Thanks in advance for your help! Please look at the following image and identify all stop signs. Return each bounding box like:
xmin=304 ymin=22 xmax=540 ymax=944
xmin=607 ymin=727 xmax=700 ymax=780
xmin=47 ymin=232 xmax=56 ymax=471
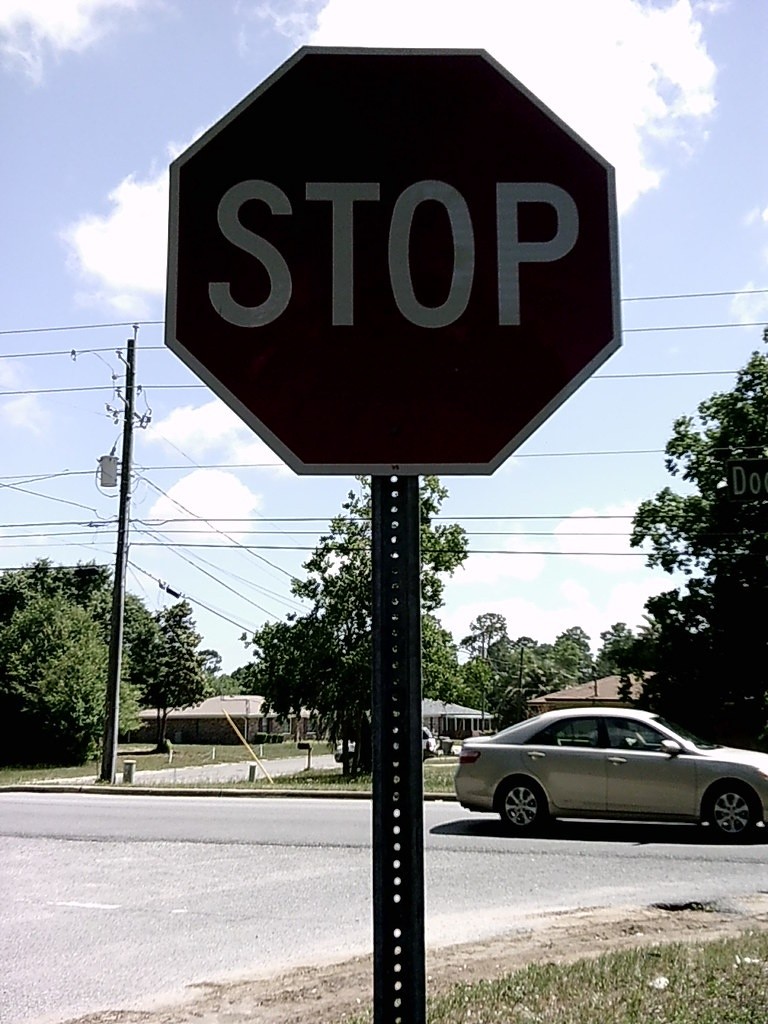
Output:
xmin=163 ymin=45 xmax=621 ymax=476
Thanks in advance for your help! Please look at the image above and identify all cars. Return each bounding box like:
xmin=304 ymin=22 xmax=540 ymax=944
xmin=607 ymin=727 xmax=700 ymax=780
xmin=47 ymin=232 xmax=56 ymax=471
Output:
xmin=454 ymin=707 xmax=768 ymax=841
